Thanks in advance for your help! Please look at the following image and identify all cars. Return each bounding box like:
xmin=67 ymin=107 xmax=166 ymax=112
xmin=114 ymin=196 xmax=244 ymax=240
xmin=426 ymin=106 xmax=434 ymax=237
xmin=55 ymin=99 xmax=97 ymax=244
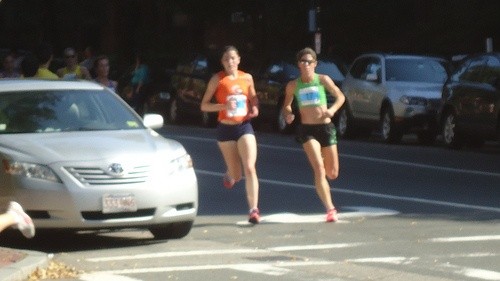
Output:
xmin=436 ymin=53 xmax=500 ymax=148
xmin=0 ymin=79 xmax=197 ymax=240
xmin=137 ymin=46 xmax=344 ymax=135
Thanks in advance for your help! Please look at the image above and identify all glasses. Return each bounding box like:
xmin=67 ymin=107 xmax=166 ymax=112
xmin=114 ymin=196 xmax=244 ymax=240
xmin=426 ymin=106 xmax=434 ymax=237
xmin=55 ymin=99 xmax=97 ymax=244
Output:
xmin=299 ymin=59 xmax=315 ymax=66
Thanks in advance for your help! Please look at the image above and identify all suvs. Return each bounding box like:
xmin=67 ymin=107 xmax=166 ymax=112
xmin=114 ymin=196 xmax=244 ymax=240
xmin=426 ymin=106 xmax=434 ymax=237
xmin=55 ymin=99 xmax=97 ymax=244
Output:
xmin=338 ymin=50 xmax=458 ymax=143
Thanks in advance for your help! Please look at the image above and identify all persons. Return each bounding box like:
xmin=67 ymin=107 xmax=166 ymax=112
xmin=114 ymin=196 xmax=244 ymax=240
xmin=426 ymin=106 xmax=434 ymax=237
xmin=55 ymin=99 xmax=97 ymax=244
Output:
xmin=0 ymin=44 xmax=200 ymax=122
xmin=284 ymin=48 xmax=345 ymax=221
xmin=201 ymin=46 xmax=259 ymax=224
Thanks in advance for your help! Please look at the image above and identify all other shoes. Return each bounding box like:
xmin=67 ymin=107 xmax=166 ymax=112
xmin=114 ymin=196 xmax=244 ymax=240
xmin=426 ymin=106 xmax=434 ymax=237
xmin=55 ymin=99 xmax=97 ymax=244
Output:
xmin=8 ymin=201 xmax=36 ymax=239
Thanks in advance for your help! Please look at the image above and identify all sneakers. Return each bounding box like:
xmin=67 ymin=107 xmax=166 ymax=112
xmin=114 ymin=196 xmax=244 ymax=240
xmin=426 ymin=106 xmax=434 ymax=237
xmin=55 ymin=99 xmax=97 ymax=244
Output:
xmin=326 ymin=207 xmax=338 ymax=221
xmin=224 ymin=174 xmax=236 ymax=189
xmin=248 ymin=208 xmax=259 ymax=222
